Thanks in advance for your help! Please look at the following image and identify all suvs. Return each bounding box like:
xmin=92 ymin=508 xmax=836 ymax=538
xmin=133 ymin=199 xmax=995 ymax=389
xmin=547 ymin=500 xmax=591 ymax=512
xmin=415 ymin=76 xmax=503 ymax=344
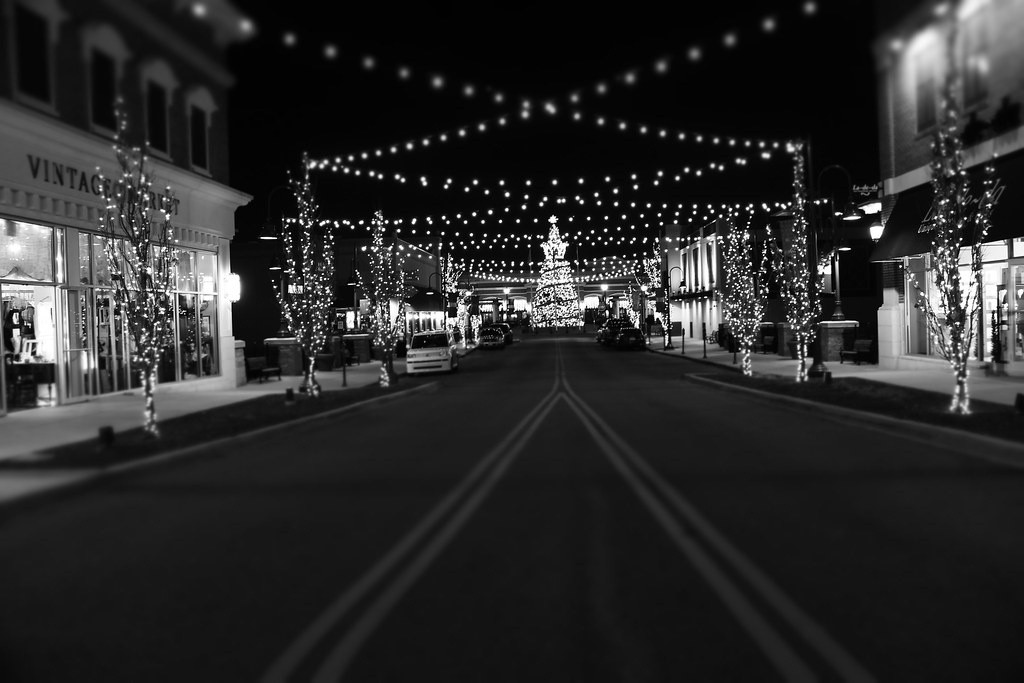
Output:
xmin=597 ymin=319 xmax=635 ymax=345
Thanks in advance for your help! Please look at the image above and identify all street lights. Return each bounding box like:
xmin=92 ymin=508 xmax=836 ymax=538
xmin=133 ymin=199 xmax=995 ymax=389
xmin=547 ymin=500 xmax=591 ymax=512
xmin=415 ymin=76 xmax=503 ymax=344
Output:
xmin=807 ymin=164 xmax=883 ymax=376
xmin=665 ymin=266 xmax=687 ymax=350
xmin=426 ymin=272 xmax=458 ymax=334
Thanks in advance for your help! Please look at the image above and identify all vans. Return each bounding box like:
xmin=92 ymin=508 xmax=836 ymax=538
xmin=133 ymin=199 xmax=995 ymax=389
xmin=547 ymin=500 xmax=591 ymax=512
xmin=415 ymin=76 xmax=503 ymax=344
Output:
xmin=406 ymin=332 xmax=460 ymax=373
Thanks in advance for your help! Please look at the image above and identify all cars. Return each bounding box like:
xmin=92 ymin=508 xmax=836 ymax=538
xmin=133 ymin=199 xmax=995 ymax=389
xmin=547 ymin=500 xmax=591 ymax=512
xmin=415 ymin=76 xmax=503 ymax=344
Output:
xmin=490 ymin=322 xmax=514 ymax=344
xmin=617 ymin=328 xmax=647 ymax=349
xmin=480 ymin=328 xmax=505 ymax=346
